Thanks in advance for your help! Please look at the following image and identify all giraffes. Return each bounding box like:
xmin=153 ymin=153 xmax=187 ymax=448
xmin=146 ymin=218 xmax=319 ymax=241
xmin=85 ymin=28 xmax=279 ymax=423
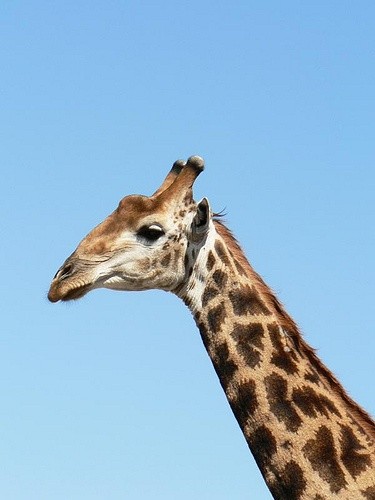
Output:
xmin=45 ymin=151 xmax=375 ymax=499
